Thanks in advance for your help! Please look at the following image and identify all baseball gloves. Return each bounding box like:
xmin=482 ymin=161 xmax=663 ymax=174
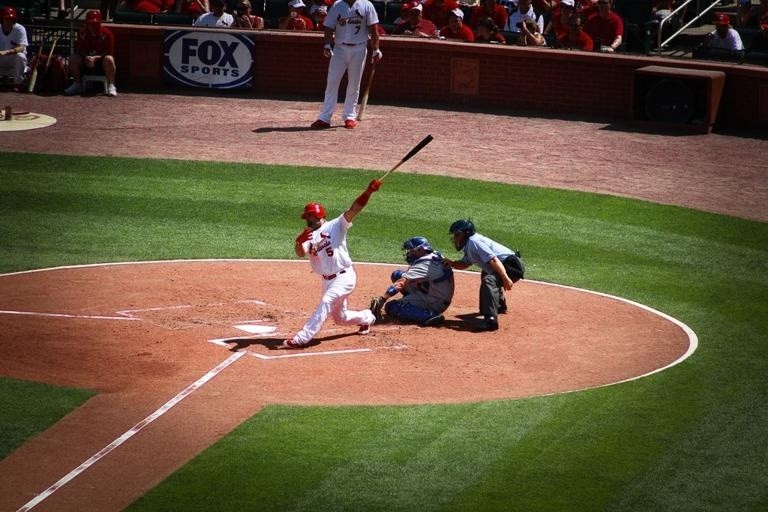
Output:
xmin=369 ymin=298 xmax=386 ymax=322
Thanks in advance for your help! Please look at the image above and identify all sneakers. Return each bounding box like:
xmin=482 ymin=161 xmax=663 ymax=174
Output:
xmin=357 ymin=317 xmax=376 ymax=335
xmin=423 ymin=312 xmax=443 ymax=326
xmin=345 ymin=119 xmax=355 ymax=129
xmin=497 ymin=304 xmax=506 ymax=314
xmin=64 ymin=83 xmax=81 ymax=94
xmin=107 ymin=85 xmax=117 ymax=97
xmin=282 ymin=339 xmax=298 ymax=349
xmin=310 ymin=119 xmax=330 ymax=130
xmin=470 ymin=317 xmax=497 ymax=332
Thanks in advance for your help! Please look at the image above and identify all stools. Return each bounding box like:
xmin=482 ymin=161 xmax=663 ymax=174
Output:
xmin=81 ymin=75 xmax=109 ymax=95
xmin=0 ymin=66 xmax=32 ymax=92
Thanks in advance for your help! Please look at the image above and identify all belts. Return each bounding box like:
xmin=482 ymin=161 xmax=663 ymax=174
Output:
xmin=323 ymin=270 xmax=344 ymax=280
xmin=342 ymin=42 xmax=364 ymax=46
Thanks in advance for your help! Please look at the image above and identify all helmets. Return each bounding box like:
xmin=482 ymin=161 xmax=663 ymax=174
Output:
xmin=449 ymin=219 xmax=475 ymax=251
xmin=300 ymin=202 xmax=325 ymax=219
xmin=399 ymin=236 xmax=433 ymax=265
xmin=86 ymin=10 xmax=102 ymax=24
xmin=0 ymin=5 xmax=17 ymax=20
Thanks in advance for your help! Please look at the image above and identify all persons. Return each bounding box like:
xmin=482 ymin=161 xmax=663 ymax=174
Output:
xmin=119 ymin=1 xmax=331 ymax=32
xmin=0 ymin=6 xmax=30 ymax=93
xmin=282 ymin=179 xmax=382 ymax=349
xmin=696 ymin=1 xmax=767 ymax=64
xmin=62 ymin=11 xmax=118 ymax=97
xmin=367 ymin=235 xmax=456 ymax=327
xmin=310 ymin=0 xmax=383 ymax=131
xmin=441 ymin=218 xmax=525 ymax=332
xmin=373 ymin=1 xmax=692 ymax=54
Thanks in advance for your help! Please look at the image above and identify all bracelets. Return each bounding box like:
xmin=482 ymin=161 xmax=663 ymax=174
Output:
xmin=386 ymin=285 xmax=398 ymax=297
xmin=324 ymin=44 xmax=331 ymax=49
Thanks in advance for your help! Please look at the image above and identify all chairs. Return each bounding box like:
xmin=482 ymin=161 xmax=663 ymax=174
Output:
xmin=745 ymin=52 xmax=768 ymax=67
xmin=152 ymin=14 xmax=193 ymax=25
xmin=692 ymin=43 xmax=712 ymax=60
xmin=381 ymin=2 xmax=402 ymax=33
xmin=541 ymin=34 xmax=557 ymax=46
xmin=373 ymin=2 xmax=385 ymax=23
xmin=115 ymin=11 xmax=151 ymax=24
xmin=500 ymin=30 xmax=520 ymax=44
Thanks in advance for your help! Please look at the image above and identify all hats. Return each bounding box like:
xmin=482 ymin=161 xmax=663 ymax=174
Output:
xmin=287 ymin=0 xmax=306 ymax=8
xmin=516 ymin=18 xmax=537 ymax=34
xmin=559 ymin=0 xmax=574 ymax=7
xmin=450 ymin=7 xmax=464 ymax=20
xmin=711 ymin=14 xmax=729 ymax=25
xmin=411 ymin=3 xmax=423 ymax=13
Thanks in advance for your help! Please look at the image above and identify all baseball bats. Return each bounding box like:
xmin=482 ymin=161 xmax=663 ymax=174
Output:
xmin=358 ymin=57 xmax=380 ymax=122
xmin=26 ymin=36 xmax=60 ymax=94
xmin=370 ymin=134 xmax=434 ymax=190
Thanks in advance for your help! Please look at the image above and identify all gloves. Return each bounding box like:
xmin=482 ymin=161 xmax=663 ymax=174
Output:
xmin=370 ymin=48 xmax=382 ymax=64
xmin=367 ymin=179 xmax=380 ymax=192
xmin=323 ymin=43 xmax=333 ymax=58
